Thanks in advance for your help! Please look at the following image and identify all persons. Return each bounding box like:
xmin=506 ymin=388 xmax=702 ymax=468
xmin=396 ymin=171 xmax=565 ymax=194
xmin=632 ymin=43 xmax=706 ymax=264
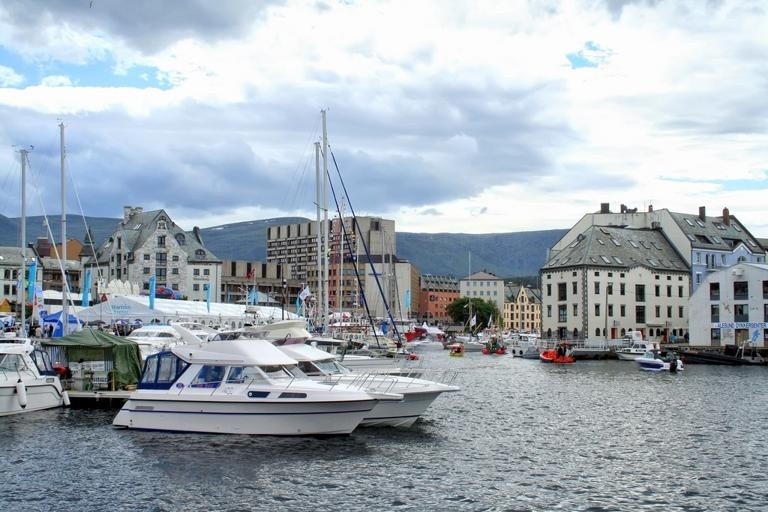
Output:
xmin=395 ymin=341 xmax=401 ymax=352
xmin=555 ymin=344 xmax=568 ymax=359
xmin=0 ymin=327 xmax=5 ymax=337
xmin=33 ymin=323 xmax=53 ymax=338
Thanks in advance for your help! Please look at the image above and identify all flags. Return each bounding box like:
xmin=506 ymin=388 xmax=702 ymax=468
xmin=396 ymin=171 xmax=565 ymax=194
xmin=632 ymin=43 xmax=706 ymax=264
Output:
xmin=471 ymin=315 xmax=476 ymax=327
xmin=486 ymin=314 xmax=492 ymax=327
xmin=298 ymin=286 xmax=310 ymax=301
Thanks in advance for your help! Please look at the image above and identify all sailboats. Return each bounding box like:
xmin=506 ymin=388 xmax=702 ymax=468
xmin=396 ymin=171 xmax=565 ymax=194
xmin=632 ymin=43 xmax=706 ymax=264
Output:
xmin=0 ymin=144 xmax=71 ymax=418
xmin=464 ymin=298 xmax=484 ymax=351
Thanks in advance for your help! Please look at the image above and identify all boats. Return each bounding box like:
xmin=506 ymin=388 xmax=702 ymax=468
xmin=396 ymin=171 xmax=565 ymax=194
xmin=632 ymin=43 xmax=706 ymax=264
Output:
xmin=0 ymin=107 xmax=461 ymax=419
xmin=110 ymin=335 xmax=404 ymax=441
xmin=447 ymin=343 xmax=465 ymax=356
xmin=275 ymin=340 xmax=463 ymax=429
xmin=482 ymin=330 xmax=763 ymax=372
